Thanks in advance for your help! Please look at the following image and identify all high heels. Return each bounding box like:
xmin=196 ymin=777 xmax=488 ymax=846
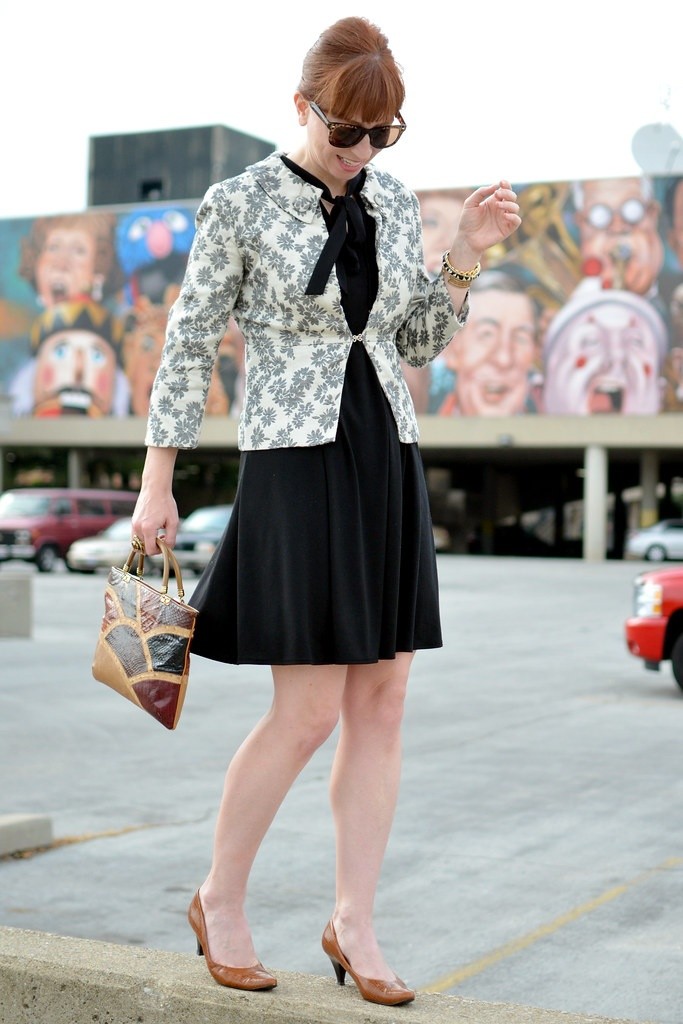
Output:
xmin=321 ymin=918 xmax=416 ymax=1005
xmin=188 ymin=889 xmax=278 ymax=991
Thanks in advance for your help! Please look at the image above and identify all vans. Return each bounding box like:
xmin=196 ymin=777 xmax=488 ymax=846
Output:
xmin=1 ymin=489 xmax=141 ymax=572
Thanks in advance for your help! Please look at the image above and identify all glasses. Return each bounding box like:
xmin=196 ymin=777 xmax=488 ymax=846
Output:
xmin=308 ymin=95 xmax=406 ymax=149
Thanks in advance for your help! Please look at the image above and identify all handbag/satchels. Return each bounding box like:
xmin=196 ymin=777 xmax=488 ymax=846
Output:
xmin=91 ymin=542 xmax=199 ymax=731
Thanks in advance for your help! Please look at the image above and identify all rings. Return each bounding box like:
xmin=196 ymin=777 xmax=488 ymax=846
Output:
xmin=132 ymin=534 xmax=145 ymax=555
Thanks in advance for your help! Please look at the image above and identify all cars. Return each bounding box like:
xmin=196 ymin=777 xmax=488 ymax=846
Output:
xmin=628 ymin=520 xmax=683 ymax=562
xmin=151 ymin=507 xmax=236 ymax=576
xmin=628 ymin=560 xmax=682 ymax=693
xmin=66 ymin=515 xmax=150 ymax=575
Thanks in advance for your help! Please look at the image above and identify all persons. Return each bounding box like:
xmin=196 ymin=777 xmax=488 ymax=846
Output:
xmin=132 ymin=18 xmax=520 ymax=1003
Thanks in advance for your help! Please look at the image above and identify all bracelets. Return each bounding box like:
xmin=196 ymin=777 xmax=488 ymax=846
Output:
xmin=442 ymin=250 xmax=481 ymax=287
